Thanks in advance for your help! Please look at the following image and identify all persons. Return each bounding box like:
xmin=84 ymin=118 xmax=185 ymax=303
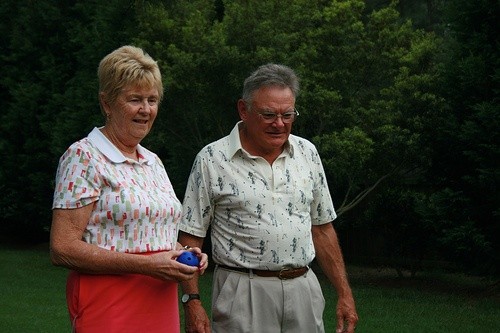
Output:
xmin=50 ymin=46 xmax=208 ymax=332
xmin=178 ymin=62 xmax=358 ymax=333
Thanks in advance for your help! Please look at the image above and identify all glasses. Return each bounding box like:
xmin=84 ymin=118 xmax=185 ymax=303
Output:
xmin=247 ymin=108 xmax=299 ymax=124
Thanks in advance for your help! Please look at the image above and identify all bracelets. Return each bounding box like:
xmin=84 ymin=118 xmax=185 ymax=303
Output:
xmin=179 ymin=246 xmax=192 ymax=250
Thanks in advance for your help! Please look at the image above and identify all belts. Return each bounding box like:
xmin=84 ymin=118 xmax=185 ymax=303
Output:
xmin=219 ymin=264 xmax=309 ymax=280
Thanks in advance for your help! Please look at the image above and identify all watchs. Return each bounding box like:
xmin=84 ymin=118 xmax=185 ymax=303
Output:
xmin=181 ymin=294 xmax=200 ymax=304
